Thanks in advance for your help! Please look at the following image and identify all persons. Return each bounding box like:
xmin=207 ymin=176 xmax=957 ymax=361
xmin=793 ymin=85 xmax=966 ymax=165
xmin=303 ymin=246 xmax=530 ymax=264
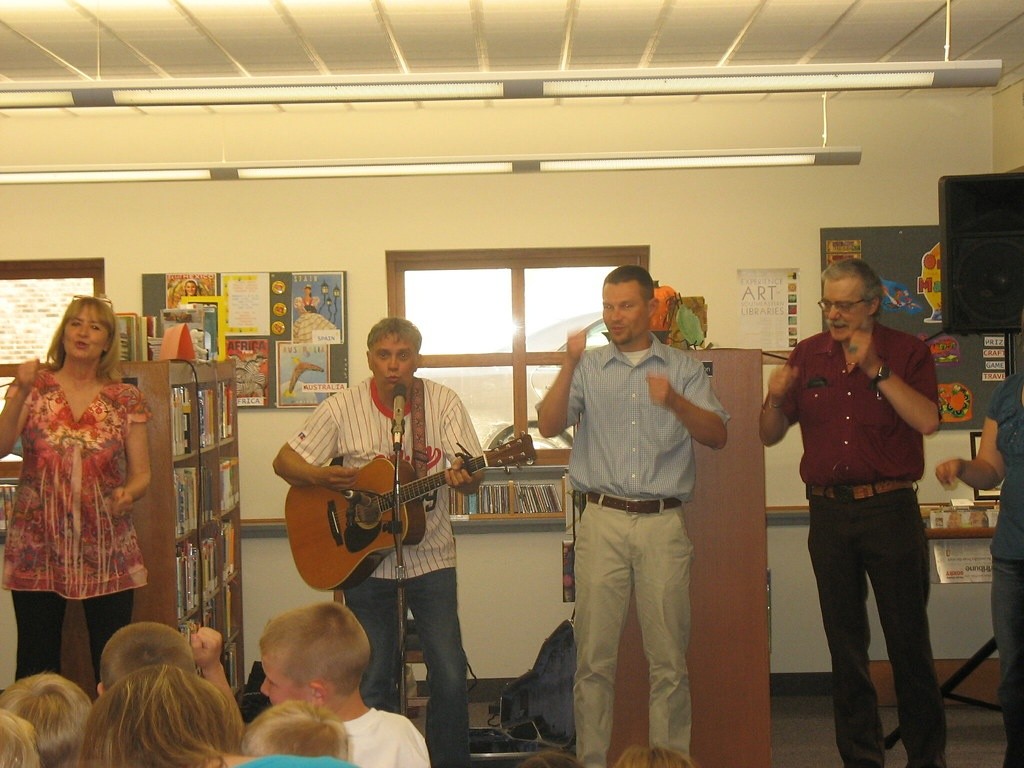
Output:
xmin=517 ymin=748 xmax=586 ymax=768
xmin=0 ymin=602 xmax=431 ymax=768
xmin=613 ymin=743 xmax=692 ymax=768
xmin=760 ymin=258 xmax=944 ymax=768
xmin=0 ymin=296 xmax=153 ymax=695
xmin=935 ymin=373 xmax=1024 ymax=768
xmin=535 ymin=265 xmax=731 ymax=759
xmin=272 ymin=319 xmax=487 ymax=768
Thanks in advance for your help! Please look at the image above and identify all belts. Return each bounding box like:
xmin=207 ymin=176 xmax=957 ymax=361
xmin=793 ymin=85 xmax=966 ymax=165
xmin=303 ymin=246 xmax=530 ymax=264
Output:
xmin=807 ymin=478 xmax=915 ymax=499
xmin=587 ymin=491 xmax=682 ymax=513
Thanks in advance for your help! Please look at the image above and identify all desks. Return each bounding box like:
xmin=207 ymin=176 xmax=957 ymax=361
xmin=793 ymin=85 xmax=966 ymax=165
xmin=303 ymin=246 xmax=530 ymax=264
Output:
xmin=884 ymin=526 xmax=1001 ymax=751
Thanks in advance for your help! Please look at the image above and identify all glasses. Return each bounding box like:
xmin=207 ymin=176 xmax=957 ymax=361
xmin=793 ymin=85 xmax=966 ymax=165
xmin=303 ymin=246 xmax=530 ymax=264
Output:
xmin=819 ymin=294 xmax=878 ymax=313
xmin=72 ymin=295 xmax=113 ymax=311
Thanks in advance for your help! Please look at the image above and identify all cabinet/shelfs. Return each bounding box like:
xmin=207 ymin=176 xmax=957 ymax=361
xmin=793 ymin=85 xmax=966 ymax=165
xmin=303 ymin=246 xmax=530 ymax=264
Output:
xmin=607 ymin=349 xmax=772 ymax=767
xmin=105 ymin=360 xmax=246 ymax=702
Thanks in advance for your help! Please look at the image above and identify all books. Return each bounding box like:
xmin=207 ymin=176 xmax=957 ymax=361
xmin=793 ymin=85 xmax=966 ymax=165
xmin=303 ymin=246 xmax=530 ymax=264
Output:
xmin=115 ymin=312 xmax=162 ymax=361
xmin=446 ymin=483 xmax=563 ymax=515
xmin=169 ymin=386 xmax=238 ymax=694
xmin=0 ymin=483 xmax=18 ymax=528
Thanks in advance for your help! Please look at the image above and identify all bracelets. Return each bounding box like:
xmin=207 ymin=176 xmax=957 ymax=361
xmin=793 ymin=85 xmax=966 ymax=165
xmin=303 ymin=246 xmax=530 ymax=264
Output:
xmin=767 ymin=399 xmax=784 ymax=408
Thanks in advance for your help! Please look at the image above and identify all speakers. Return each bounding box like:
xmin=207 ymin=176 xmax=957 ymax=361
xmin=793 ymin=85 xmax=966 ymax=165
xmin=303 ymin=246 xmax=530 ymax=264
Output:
xmin=939 ymin=172 xmax=1023 ymax=334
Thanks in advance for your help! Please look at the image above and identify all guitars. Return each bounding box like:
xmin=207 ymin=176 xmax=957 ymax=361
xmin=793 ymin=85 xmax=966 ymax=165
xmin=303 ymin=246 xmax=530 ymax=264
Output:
xmin=285 ymin=432 xmax=537 ymax=591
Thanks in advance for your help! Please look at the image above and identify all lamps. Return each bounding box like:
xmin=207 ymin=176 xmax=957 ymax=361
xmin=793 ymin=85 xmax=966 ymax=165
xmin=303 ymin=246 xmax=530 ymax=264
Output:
xmin=0 ymin=1 xmax=1003 ymax=185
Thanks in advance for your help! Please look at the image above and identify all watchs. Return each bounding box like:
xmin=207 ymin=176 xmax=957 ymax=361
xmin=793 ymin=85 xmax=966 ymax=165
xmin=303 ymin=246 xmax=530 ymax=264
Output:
xmin=871 ymin=364 xmax=891 ymax=384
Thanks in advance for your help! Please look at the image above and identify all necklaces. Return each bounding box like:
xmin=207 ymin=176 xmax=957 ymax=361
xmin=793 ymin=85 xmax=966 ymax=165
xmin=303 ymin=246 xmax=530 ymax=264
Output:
xmin=846 ymin=362 xmax=855 ymax=365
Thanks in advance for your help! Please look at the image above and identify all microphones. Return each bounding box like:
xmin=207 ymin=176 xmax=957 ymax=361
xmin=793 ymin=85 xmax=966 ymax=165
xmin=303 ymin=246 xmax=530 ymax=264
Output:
xmin=390 ymin=382 xmax=406 ymax=452
